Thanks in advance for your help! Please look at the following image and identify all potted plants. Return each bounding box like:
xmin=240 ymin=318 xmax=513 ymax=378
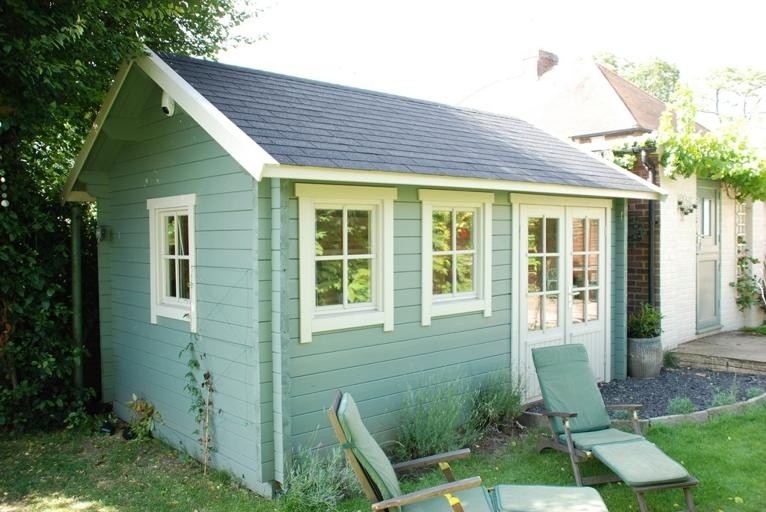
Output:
xmin=727 ymin=255 xmax=766 ymax=329
xmin=628 ymin=301 xmax=667 ymax=378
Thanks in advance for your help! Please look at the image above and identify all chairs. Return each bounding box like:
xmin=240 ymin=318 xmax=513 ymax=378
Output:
xmin=327 ymin=388 xmax=609 ymax=512
xmin=531 ymin=342 xmax=700 ymax=511
